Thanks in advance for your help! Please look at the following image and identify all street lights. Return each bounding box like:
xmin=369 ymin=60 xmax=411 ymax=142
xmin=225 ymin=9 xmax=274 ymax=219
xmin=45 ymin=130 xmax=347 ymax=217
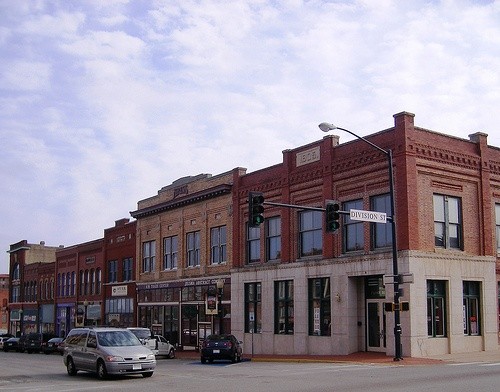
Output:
xmin=84 ymin=298 xmax=89 ymax=326
xmin=216 ymin=278 xmax=224 ymax=336
xmin=317 ymin=121 xmax=404 ymax=362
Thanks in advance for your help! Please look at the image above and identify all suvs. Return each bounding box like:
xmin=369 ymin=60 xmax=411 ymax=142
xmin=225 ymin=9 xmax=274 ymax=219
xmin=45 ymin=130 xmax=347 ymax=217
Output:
xmin=126 ymin=326 xmax=158 ymax=356
xmin=24 ymin=332 xmax=59 ymax=354
xmin=63 ymin=326 xmax=157 ymax=380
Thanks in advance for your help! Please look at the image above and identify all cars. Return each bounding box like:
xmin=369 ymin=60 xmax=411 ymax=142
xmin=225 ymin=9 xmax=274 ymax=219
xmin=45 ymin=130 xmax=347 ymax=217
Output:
xmin=200 ymin=333 xmax=243 ymax=364
xmin=153 ymin=334 xmax=176 ymax=360
xmin=44 ymin=338 xmax=65 ymax=356
xmin=0 ymin=337 xmax=25 ymax=353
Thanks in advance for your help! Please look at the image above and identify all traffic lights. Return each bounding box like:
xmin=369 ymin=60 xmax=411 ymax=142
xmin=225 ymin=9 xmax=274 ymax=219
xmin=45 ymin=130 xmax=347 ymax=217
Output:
xmin=385 ymin=302 xmax=395 ymax=312
xmin=400 ymin=300 xmax=409 ymax=312
xmin=249 ymin=191 xmax=264 ymax=228
xmin=324 ymin=199 xmax=340 ymax=235
